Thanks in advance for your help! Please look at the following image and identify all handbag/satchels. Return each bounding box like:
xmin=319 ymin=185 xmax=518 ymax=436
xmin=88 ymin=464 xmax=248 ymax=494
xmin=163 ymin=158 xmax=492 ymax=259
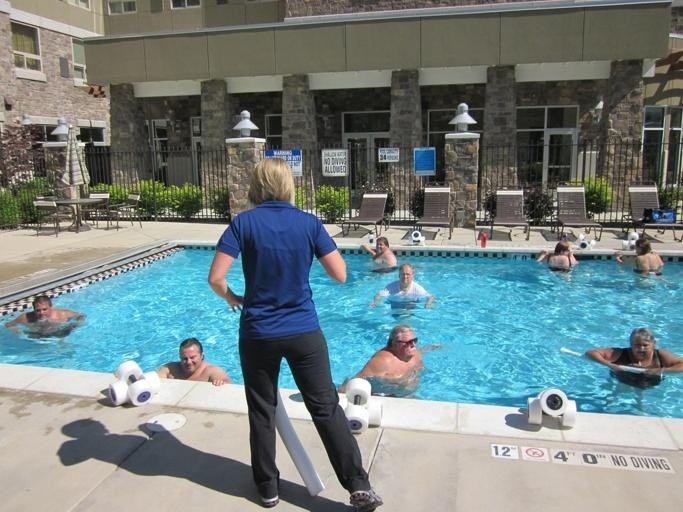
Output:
xmin=642 ymin=208 xmax=676 ymax=224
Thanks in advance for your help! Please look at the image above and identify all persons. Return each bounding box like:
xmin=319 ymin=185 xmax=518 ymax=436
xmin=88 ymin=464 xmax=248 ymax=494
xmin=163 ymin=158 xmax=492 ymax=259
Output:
xmin=586 ymin=328 xmax=683 ymax=375
xmin=4 ymin=295 xmax=84 ymax=339
xmin=368 ymin=263 xmax=433 ymax=318
xmin=208 ymin=157 xmax=383 ymax=511
xmin=537 ymin=241 xmax=578 ymax=281
xmin=615 ymin=238 xmax=665 ymax=279
xmin=361 ymin=236 xmax=398 ymax=273
xmin=155 ymin=338 xmax=232 ymax=386
xmin=334 ymin=325 xmax=440 ymax=399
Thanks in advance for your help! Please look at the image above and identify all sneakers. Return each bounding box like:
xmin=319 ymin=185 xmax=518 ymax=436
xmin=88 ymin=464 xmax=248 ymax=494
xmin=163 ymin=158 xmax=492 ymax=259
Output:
xmin=350 ymin=487 xmax=383 ymax=512
xmin=261 ymin=494 xmax=279 ymax=507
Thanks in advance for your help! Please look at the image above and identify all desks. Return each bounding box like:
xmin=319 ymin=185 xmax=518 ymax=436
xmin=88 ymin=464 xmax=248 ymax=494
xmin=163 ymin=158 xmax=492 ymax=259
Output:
xmin=55 ymin=198 xmax=103 ymax=232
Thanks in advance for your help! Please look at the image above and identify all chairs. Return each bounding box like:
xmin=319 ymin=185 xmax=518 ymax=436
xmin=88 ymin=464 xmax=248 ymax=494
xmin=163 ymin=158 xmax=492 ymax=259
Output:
xmin=551 ymin=181 xmax=603 ymax=241
xmin=622 ymin=180 xmax=683 ymax=242
xmin=35 ymin=194 xmax=57 ymax=224
xmin=416 ymin=182 xmax=454 ymax=240
xmin=342 ymin=190 xmax=390 ymax=238
xmin=85 ymin=192 xmax=112 ymax=229
xmin=110 ymin=193 xmax=142 ymax=231
xmin=490 ymin=185 xmax=529 ymax=241
xmin=33 ymin=200 xmax=60 ymax=237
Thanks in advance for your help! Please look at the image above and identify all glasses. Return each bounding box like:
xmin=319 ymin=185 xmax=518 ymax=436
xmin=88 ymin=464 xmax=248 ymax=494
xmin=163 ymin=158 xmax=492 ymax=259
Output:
xmin=398 ymin=338 xmax=418 ymax=345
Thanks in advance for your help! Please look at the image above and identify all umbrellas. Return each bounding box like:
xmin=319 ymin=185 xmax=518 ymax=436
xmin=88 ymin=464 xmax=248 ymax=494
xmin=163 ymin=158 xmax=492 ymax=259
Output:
xmin=61 ymin=125 xmax=91 ymax=224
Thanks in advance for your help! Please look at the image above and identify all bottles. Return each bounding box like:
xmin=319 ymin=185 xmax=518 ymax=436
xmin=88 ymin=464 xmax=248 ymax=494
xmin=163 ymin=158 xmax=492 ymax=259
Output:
xmin=481 ymin=231 xmax=487 ymax=248
